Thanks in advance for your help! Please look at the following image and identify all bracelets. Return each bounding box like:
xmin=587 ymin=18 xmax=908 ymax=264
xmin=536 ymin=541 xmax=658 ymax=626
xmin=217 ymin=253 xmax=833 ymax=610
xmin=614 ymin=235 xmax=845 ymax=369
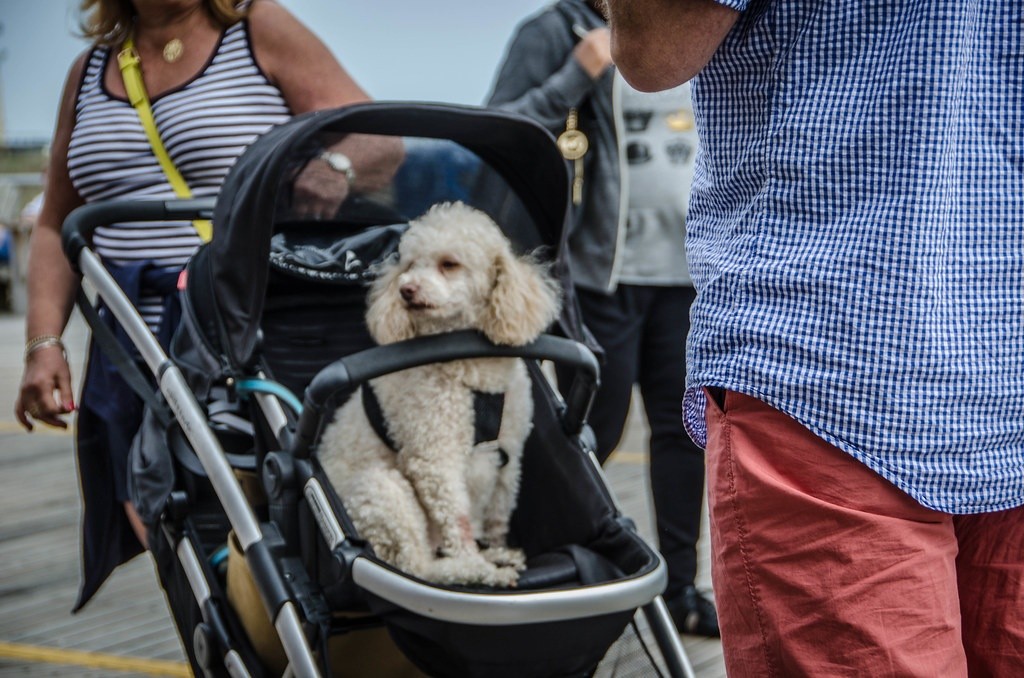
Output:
xmin=23 ymin=333 xmax=67 ymax=361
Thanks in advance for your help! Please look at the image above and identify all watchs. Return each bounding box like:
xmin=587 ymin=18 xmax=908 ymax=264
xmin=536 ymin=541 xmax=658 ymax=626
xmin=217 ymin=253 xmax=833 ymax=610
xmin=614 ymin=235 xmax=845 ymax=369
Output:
xmin=319 ymin=153 xmax=356 ymax=183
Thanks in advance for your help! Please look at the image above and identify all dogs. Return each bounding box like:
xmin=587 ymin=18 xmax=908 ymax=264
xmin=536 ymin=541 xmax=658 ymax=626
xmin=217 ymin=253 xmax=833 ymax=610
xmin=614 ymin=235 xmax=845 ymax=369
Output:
xmin=313 ymin=196 xmax=565 ymax=592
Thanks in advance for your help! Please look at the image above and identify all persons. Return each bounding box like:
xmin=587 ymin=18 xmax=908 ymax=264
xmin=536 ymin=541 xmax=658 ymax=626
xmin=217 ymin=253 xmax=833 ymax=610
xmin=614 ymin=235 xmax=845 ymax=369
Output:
xmin=483 ymin=0 xmax=720 ymax=638
xmin=606 ymin=0 xmax=1023 ymax=678
xmin=14 ymin=0 xmax=404 ymax=614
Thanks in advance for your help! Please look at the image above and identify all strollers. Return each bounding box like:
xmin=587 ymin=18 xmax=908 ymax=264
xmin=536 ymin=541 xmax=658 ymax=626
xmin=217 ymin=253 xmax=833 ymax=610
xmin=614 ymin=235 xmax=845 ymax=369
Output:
xmin=61 ymin=101 xmax=694 ymax=678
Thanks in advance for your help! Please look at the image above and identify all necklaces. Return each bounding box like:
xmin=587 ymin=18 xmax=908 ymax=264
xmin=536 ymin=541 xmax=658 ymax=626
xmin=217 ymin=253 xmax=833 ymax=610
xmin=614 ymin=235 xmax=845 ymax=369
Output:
xmin=160 ymin=37 xmax=183 ymax=64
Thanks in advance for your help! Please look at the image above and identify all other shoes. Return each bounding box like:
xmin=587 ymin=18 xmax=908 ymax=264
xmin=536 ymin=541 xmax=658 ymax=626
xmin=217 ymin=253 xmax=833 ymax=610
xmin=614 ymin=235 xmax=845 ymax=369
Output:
xmin=667 ymin=586 xmax=718 ymax=638
